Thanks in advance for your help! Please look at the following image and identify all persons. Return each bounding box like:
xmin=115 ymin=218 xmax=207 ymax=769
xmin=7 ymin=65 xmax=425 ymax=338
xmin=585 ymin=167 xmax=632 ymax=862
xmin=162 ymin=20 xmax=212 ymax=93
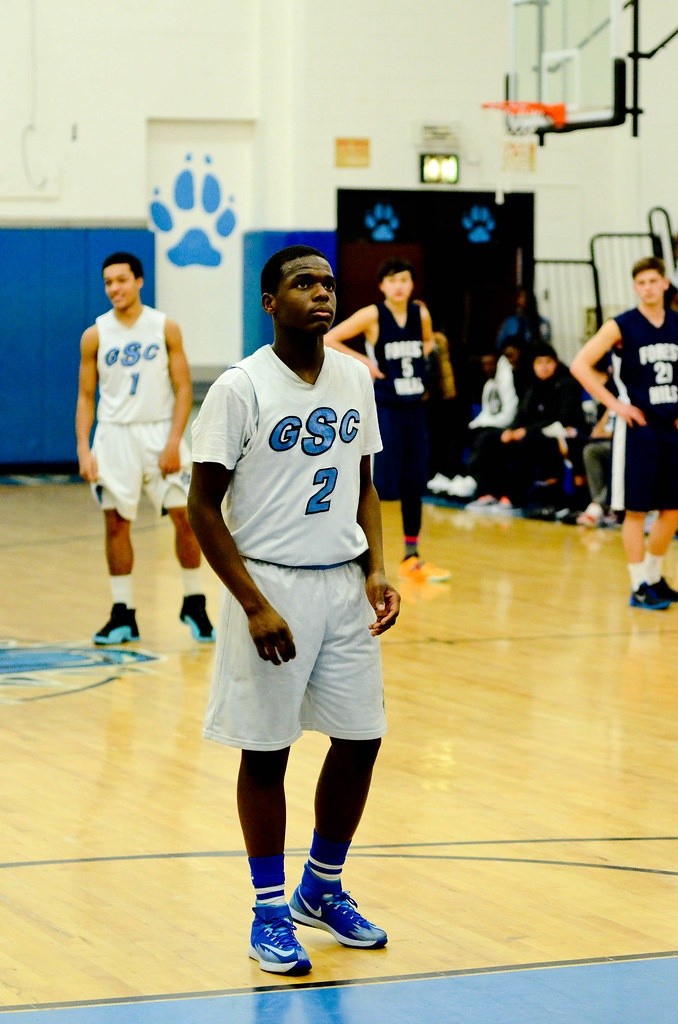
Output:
xmin=427 ymin=284 xmax=678 ymax=540
xmin=322 ymin=259 xmax=450 ymax=583
xmin=187 ymin=246 xmax=401 ymax=974
xmin=570 ymin=258 xmax=678 ymax=610
xmin=76 ymin=253 xmax=216 ymax=644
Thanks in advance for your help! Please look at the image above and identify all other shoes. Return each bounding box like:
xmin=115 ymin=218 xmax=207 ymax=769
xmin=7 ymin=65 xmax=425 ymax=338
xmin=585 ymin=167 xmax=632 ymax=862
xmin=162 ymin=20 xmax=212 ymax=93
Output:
xmin=576 ymin=501 xmax=603 ymax=529
xmin=464 ymin=496 xmax=513 ymax=515
xmin=398 ymin=554 xmax=451 ymax=583
xmin=427 ymin=472 xmax=479 ymax=498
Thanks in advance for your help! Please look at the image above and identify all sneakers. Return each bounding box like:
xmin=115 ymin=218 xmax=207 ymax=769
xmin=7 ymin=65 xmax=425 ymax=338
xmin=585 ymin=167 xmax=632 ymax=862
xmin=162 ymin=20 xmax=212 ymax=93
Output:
xmin=180 ymin=593 xmax=216 ymax=643
xmin=630 ymin=576 xmax=678 ymax=611
xmin=248 ymin=899 xmax=312 ymax=977
xmin=92 ymin=604 xmax=141 ymax=644
xmin=289 ymin=882 xmax=389 ymax=950
